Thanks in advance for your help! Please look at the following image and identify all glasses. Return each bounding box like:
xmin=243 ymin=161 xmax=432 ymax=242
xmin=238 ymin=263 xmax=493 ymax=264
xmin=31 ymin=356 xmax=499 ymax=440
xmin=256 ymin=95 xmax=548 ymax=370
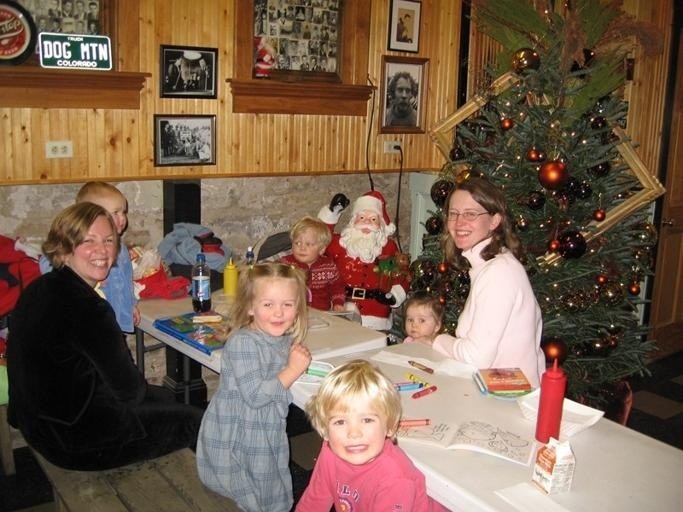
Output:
xmin=445 ymin=208 xmax=493 ymax=221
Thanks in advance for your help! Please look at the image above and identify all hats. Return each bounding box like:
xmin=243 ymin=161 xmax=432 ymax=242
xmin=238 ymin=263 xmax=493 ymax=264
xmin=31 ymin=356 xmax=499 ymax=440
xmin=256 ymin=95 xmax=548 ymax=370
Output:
xmin=354 ymin=191 xmax=397 ymax=235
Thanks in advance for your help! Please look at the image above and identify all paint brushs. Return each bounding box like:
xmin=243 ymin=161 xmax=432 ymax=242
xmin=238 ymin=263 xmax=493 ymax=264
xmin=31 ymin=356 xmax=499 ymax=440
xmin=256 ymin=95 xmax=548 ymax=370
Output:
xmin=392 ymin=381 xmax=416 ymax=387
xmin=411 ymin=385 xmax=438 ymax=398
xmin=303 ymin=368 xmax=328 ymax=378
xmin=394 ymin=383 xmax=423 ymax=392
xmin=408 ymin=360 xmax=434 ymax=374
xmin=398 ymin=419 xmax=430 ymax=426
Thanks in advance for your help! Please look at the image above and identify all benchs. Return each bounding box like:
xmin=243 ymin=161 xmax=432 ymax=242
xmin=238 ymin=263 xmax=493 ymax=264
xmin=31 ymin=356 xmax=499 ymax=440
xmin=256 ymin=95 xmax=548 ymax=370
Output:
xmin=29 ymin=446 xmax=247 ymax=512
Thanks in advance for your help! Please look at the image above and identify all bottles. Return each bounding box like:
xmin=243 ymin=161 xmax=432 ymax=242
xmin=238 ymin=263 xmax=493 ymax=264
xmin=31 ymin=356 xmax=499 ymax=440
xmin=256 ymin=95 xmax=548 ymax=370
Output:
xmin=535 ymin=359 xmax=567 ymax=444
xmin=246 ymin=247 xmax=253 ymax=265
xmin=191 ymin=254 xmax=211 ymax=313
xmin=222 ymin=255 xmax=238 ymax=296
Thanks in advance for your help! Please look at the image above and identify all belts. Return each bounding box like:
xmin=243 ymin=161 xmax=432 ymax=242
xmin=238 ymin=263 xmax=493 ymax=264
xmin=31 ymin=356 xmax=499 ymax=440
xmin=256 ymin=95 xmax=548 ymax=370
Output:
xmin=344 ymin=286 xmax=383 ymax=300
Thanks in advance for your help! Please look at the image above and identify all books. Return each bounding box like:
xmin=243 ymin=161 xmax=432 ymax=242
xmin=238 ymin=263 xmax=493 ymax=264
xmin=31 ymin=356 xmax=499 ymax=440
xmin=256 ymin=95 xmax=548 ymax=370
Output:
xmin=478 ymin=368 xmax=532 ymax=391
xmin=397 ymin=416 xmax=535 ymax=466
xmin=473 ymin=372 xmax=529 ymax=395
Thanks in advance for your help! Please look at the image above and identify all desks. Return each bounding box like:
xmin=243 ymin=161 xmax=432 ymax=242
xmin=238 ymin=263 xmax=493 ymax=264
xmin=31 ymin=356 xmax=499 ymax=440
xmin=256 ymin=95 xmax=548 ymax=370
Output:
xmin=289 ymin=341 xmax=683 ymax=512
xmin=134 ymin=287 xmax=387 ymax=406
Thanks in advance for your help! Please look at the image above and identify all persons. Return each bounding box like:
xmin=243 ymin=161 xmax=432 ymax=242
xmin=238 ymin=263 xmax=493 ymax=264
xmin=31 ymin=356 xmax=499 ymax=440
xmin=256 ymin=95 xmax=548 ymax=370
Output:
xmin=386 ymin=72 xmax=418 ymax=127
xmin=197 ymin=262 xmax=314 ymax=512
xmin=88 ymin=22 xmax=98 ymax=35
xmin=50 ymin=19 xmax=62 ymax=32
xmin=62 ymin=1 xmax=73 ymax=16
xmin=397 ymin=15 xmax=413 ymax=43
xmin=48 ymin=0 xmax=61 ymax=17
xmin=75 ymin=1 xmax=86 ymax=19
xmin=87 ymin=2 xmax=99 ymax=20
xmin=268 ymin=0 xmax=339 ymax=72
xmin=165 ymin=57 xmax=213 ymax=92
xmin=162 ymin=123 xmax=211 ymax=160
xmin=403 ymin=294 xmax=444 ymax=343
xmin=37 ymin=17 xmax=46 ymax=33
xmin=295 ymin=360 xmax=450 ymax=511
xmin=274 ymin=217 xmax=346 ymax=312
xmin=40 ymin=181 xmax=141 ymax=332
xmin=253 ymin=37 xmax=275 ymax=79
xmin=413 ymin=177 xmax=545 ymax=388
xmin=76 ymin=22 xmax=86 ymax=35
xmin=317 ymin=191 xmax=414 ymax=330
xmin=7 ymin=203 xmax=205 ymax=471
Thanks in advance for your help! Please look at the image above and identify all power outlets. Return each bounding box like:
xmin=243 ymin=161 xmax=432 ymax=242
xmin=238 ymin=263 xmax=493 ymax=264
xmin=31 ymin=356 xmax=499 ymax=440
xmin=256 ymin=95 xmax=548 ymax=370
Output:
xmin=45 ymin=141 xmax=73 ymax=159
xmin=383 ymin=142 xmax=402 ymax=154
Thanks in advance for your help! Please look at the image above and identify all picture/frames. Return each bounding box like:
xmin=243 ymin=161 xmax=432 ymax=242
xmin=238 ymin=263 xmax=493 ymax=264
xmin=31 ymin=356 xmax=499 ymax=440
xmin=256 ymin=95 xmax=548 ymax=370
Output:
xmin=387 ymin=0 xmax=421 ymax=53
xmin=378 ymin=54 xmax=430 ymax=134
xmin=154 ymin=114 xmax=216 ymax=166
xmin=159 ymin=44 xmax=219 ymax=99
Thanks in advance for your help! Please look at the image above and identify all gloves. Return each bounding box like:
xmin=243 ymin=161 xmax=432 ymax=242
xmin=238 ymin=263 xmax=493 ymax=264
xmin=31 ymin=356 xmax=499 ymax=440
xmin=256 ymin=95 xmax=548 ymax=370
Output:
xmin=374 ymin=289 xmax=396 ymax=306
xmin=329 ymin=192 xmax=351 ymax=213
